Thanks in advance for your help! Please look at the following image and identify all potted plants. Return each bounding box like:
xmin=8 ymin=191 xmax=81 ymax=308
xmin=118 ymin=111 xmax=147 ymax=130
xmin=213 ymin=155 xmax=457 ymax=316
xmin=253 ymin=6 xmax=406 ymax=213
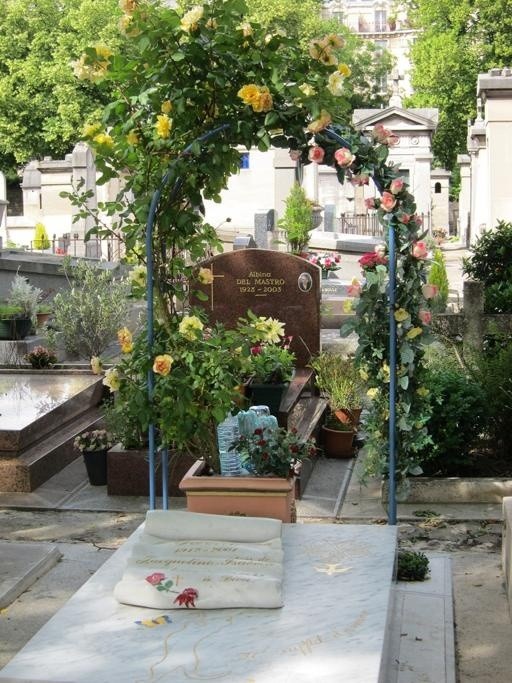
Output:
xmin=302 ymin=340 xmax=374 ymax=459
xmin=0 ymin=264 xmax=53 ymax=340
xmin=72 ymin=428 xmax=109 ymax=485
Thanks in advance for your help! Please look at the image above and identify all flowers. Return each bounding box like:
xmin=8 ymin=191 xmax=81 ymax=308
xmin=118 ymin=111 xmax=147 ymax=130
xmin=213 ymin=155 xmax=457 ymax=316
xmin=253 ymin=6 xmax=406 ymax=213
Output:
xmin=304 ymin=250 xmax=343 ymax=271
xmin=227 ymin=425 xmax=325 ymax=478
